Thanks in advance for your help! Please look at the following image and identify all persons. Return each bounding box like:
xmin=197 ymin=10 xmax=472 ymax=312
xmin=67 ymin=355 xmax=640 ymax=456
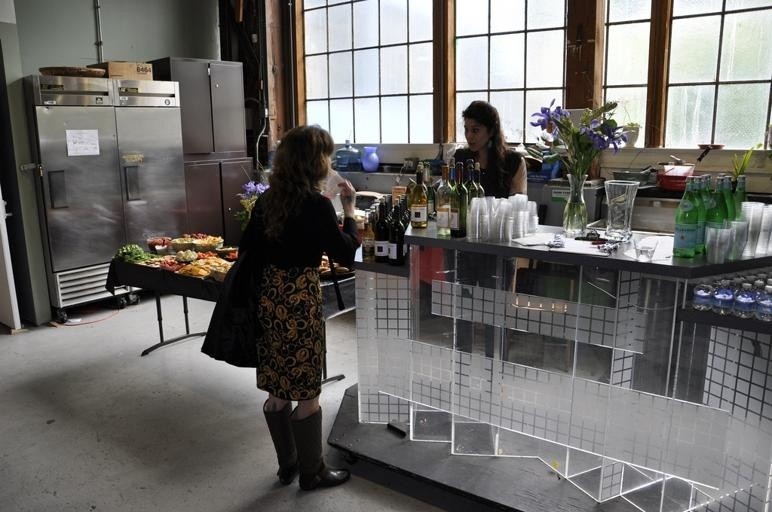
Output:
xmin=201 ymin=126 xmax=360 ymax=492
xmin=450 ymin=101 xmax=527 ymax=394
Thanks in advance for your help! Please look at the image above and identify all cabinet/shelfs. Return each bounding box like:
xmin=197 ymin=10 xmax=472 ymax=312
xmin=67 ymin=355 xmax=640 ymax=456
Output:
xmin=185 ymin=157 xmax=253 ymax=244
xmin=147 ymin=55 xmax=247 ymax=160
xmin=328 ymin=205 xmax=772 ymax=511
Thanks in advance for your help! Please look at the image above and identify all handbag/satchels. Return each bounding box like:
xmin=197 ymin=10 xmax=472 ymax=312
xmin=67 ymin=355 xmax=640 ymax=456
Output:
xmin=199 ymin=260 xmax=261 ymax=369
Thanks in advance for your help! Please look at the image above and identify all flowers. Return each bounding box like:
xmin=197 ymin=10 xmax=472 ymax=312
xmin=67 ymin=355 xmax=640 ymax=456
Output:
xmin=525 ymin=99 xmax=641 ymax=173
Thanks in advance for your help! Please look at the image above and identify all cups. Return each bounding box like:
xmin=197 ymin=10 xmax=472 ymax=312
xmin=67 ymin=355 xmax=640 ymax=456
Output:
xmin=705 ymin=202 xmax=772 ymax=264
xmin=605 ymin=180 xmax=640 ymax=242
xmin=467 ymin=194 xmax=538 ymax=242
xmin=634 ymin=237 xmax=658 ymax=262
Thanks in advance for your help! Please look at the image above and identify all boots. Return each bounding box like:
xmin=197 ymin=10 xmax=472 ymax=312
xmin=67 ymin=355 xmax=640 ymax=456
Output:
xmin=262 ymin=398 xmax=300 ymax=487
xmin=288 ymin=404 xmax=351 ymax=491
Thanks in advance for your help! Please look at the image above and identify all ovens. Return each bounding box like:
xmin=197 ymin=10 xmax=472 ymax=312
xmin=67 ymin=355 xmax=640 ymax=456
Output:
xmin=113 ymin=78 xmax=189 ymax=298
xmin=22 ymin=75 xmax=130 ymax=317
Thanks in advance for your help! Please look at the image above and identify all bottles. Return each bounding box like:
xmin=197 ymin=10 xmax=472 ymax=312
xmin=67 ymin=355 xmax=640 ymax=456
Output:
xmin=388 ymin=198 xmax=407 ymax=266
xmin=724 ymin=176 xmax=736 ymax=221
xmin=733 ymin=283 xmax=757 ymax=319
xmin=404 ymin=194 xmax=411 ymax=215
xmin=474 ymin=163 xmax=484 ymax=197
xmin=448 ymin=158 xmax=455 ymax=187
xmin=705 ymin=174 xmax=711 ymax=201
xmin=401 ymin=200 xmax=410 ymax=223
xmin=401 ymin=194 xmax=411 ymax=219
xmin=436 ymin=164 xmax=452 ymax=237
xmin=371 ymin=207 xmax=376 ymax=234
xmin=373 ymin=204 xmax=378 ymax=225
xmin=411 ymin=165 xmax=428 ymax=228
xmin=464 ymin=159 xmax=478 ymax=206
xmin=362 ymin=209 xmax=374 ymax=256
xmin=383 ymin=196 xmax=392 ymax=224
xmin=387 ymin=195 xmax=392 ymax=221
xmin=375 ymin=198 xmax=391 ymax=263
xmin=702 ymin=175 xmax=710 ymax=213
xmin=713 ymin=280 xmax=735 ymax=315
xmin=692 ymin=283 xmax=714 ymax=311
xmin=673 ymin=176 xmax=699 ymax=258
xmin=735 ymin=175 xmax=748 ymax=220
xmin=396 ymin=195 xmax=408 ymax=230
xmin=732 ymin=278 xmax=742 ymax=301
xmin=705 ymin=177 xmax=728 ymax=257
xmin=424 ymin=162 xmax=434 ymax=218
xmin=450 ymin=162 xmax=468 ymax=238
xmin=695 ymin=177 xmax=705 ymax=254
xmin=752 ymin=280 xmax=766 ymax=303
xmin=755 ymin=285 xmax=772 ymax=322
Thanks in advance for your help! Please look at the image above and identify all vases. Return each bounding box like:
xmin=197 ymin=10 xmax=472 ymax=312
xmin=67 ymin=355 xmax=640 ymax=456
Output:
xmin=561 ymin=174 xmax=589 ymax=237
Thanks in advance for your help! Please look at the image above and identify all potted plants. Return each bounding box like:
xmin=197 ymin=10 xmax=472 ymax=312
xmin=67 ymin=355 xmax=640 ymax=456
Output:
xmin=728 ymin=146 xmax=755 ymax=193
xmin=623 ymin=122 xmax=640 ymax=149
